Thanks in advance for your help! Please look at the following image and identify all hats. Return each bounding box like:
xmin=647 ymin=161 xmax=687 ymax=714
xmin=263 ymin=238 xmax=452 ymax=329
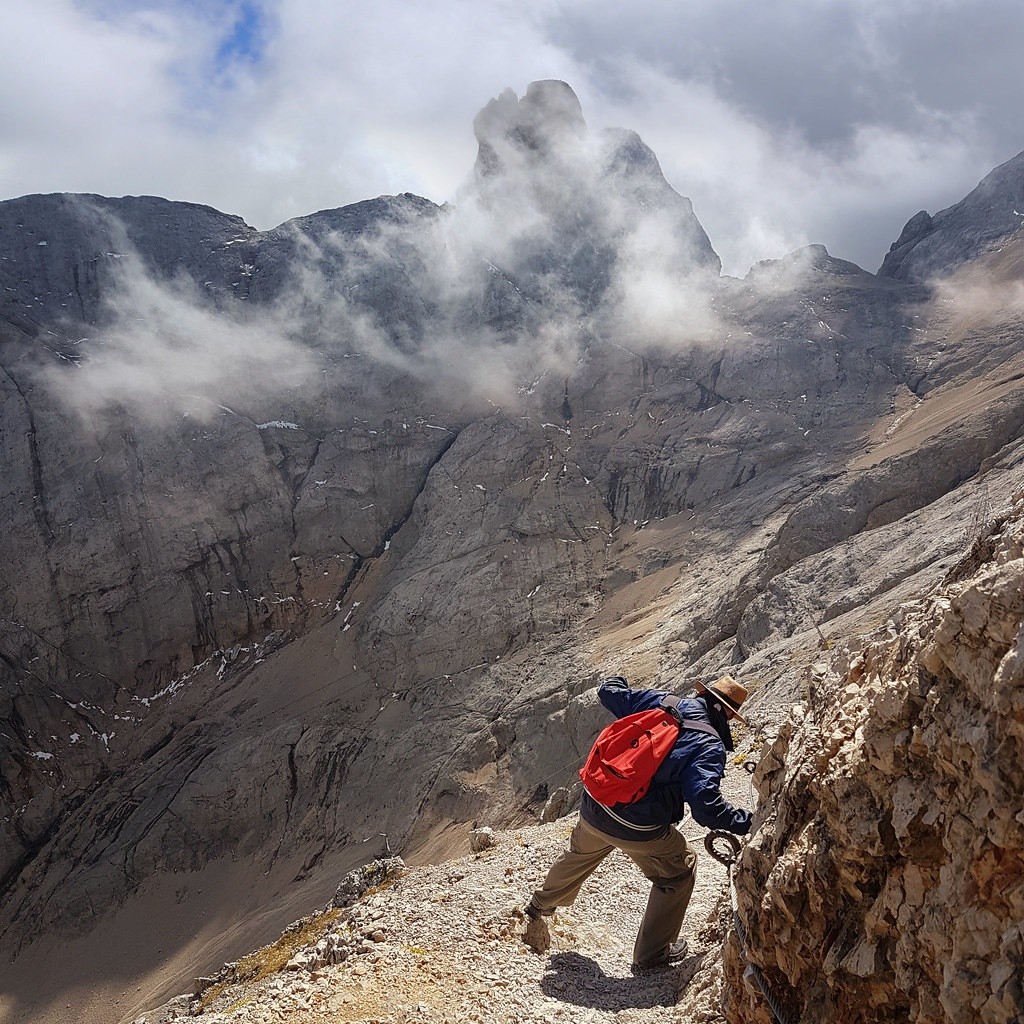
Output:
xmin=693 ymin=674 xmax=750 ymax=726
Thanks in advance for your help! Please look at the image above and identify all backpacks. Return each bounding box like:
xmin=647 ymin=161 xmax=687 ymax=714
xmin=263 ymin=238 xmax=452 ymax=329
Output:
xmin=577 ymin=694 xmax=724 ymax=812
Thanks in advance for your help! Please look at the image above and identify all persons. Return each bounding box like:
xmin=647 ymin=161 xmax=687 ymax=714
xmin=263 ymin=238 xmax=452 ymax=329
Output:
xmin=523 ymin=671 xmax=758 ymax=974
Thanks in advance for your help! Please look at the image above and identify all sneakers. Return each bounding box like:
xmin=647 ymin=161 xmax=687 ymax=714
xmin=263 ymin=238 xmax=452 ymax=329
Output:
xmin=523 ymin=900 xmax=556 ymax=921
xmin=664 ymin=938 xmax=689 ymax=963
